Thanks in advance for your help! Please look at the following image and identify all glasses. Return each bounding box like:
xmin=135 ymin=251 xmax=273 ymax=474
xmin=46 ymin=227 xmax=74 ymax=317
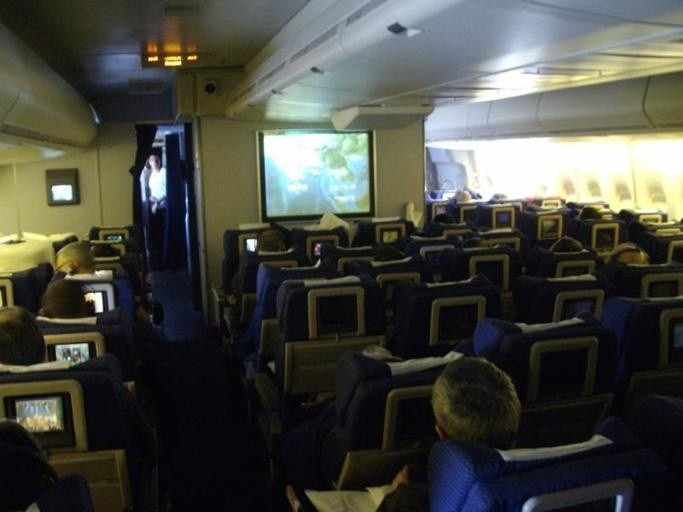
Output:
xmin=54 ymin=259 xmax=72 ymax=273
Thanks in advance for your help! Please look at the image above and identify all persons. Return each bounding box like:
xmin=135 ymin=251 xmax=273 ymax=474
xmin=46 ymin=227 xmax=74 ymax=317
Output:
xmin=91 ymin=239 xmax=143 ymax=294
xmin=0 ymin=421 xmax=95 ymax=512
xmin=373 ymin=357 xmax=521 ymax=511
xmin=55 ymin=242 xmax=152 ymax=322
xmin=36 ymin=278 xmax=95 ymax=319
xmin=140 ymin=151 xmax=172 ymax=252
xmin=0 ymin=305 xmax=157 ymax=459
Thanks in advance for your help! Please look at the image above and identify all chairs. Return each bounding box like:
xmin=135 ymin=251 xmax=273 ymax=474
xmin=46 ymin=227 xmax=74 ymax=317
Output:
xmin=290 ymin=227 xmax=349 ymax=264
xmin=463 ymin=232 xmax=529 ymax=251
xmin=35 ymin=306 xmax=144 ymax=400
xmin=475 ymin=205 xmax=519 ymax=229
xmin=48 ymin=263 xmax=154 ymax=327
xmin=286 ymin=415 xmax=670 ymax=511
xmin=655 ymin=236 xmax=682 ymax=262
xmin=574 ymin=219 xmax=628 ymax=260
xmin=211 ymin=223 xmax=289 ymax=328
xmin=605 ymin=262 xmax=682 ymax=298
xmin=221 ymin=247 xmax=312 ymax=351
xmin=302 ymin=338 xmax=465 ymax=489
xmin=388 ymin=273 xmax=503 ymax=359
xmin=437 ymin=247 xmax=522 ymax=314
xmin=456 ymin=310 xmax=613 ymax=449
xmin=314 ymin=241 xmax=384 ymax=268
xmin=254 ymin=273 xmax=384 ymax=479
xmin=342 ymin=256 xmax=434 ymax=317
xmin=522 ymin=211 xmax=579 ymax=249
xmin=90 ymin=244 xmax=151 ymax=282
xmin=428 ymin=221 xmax=471 ymax=238
xmin=89 ymin=227 xmax=139 ymax=240
xmin=0 ymin=276 xmax=37 ymax=311
xmin=398 ymin=233 xmax=461 ymax=260
xmin=446 ymin=204 xmax=477 ymax=227
xmin=0 ymin=369 xmax=159 ymax=512
xmin=230 ymin=257 xmax=336 ymax=424
xmin=525 ymin=245 xmax=603 ymax=279
xmin=507 ymin=274 xmax=605 ymax=322
xmin=605 ymin=242 xmax=649 ymax=265
xmin=91 ymin=253 xmax=152 ymax=307
xmin=603 ymin=296 xmax=683 ymax=414
xmin=351 ymin=217 xmax=414 ymax=247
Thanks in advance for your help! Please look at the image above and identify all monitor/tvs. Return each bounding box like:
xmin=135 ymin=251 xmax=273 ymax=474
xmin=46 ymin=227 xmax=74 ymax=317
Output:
xmin=307 ymin=287 xmax=365 ymax=340
xmin=667 ymin=240 xmax=683 ymax=267
xmin=654 ymin=228 xmax=680 ymax=236
xmin=82 ymin=283 xmax=116 ymax=313
xmin=527 ymin=336 xmax=599 ymax=404
xmin=552 ymin=288 xmax=604 ymax=323
xmin=419 ymin=245 xmax=452 ymax=259
xmin=94 ymin=263 xmax=123 ymax=277
xmin=583 ymin=205 xmax=604 ymax=212
xmin=431 ymin=201 xmax=447 ymax=220
xmin=0 ymin=379 xmax=89 ymax=455
xmin=641 ymin=273 xmax=683 ymax=299
xmin=488 ymin=237 xmax=521 ymax=255
xmin=238 ymin=233 xmax=258 ymax=255
xmin=504 ymin=202 xmax=522 ymax=212
xmin=492 ymin=207 xmax=515 ymax=229
xmin=638 ymin=214 xmax=662 ymax=225
xmin=521 ymin=478 xmax=634 ymax=512
xmin=382 ymin=384 xmax=436 ymax=450
xmin=307 ymin=235 xmax=340 ymax=257
xmin=468 ymin=253 xmax=510 ymax=291
xmin=89 ymin=244 xmax=126 ymax=256
xmin=660 ymin=308 xmax=683 ymax=366
xmin=442 ymin=192 xmax=455 ymax=201
xmin=375 ymin=223 xmax=405 ymax=243
xmin=537 ymin=215 xmax=563 ymax=241
xmin=338 ymin=257 xmax=373 ymax=277
xmin=43 ymin=332 xmax=104 ymax=365
xmin=592 ymin=222 xmax=620 ymax=257
xmin=376 ymin=272 xmax=421 ymax=288
xmin=555 ymin=260 xmax=595 ymax=277
xmin=540 ymin=200 xmax=560 ymax=209
xmin=429 ymin=295 xmax=485 ymax=345
xmin=98 ymin=228 xmax=130 ymax=243
xmin=460 ymin=205 xmax=476 ymax=222
xmin=0 ymin=279 xmax=15 ymax=308
